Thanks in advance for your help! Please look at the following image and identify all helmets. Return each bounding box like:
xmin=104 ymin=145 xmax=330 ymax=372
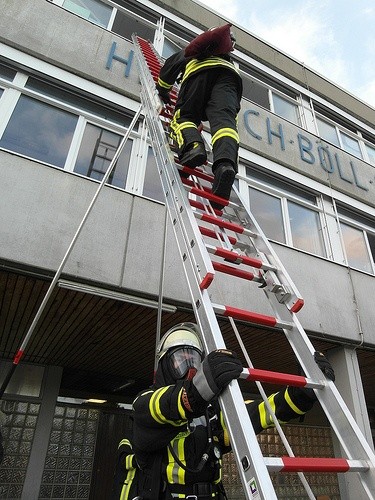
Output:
xmin=156 ymin=322 xmax=205 ymax=387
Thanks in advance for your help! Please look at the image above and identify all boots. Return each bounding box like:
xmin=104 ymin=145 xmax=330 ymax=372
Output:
xmin=210 ymin=160 xmax=235 ymax=210
xmin=177 ymin=142 xmax=207 ymax=178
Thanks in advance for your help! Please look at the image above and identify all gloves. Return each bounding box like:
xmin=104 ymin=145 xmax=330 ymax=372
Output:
xmin=192 ymin=348 xmax=243 ymax=402
xmin=295 ymin=351 xmax=336 ymax=401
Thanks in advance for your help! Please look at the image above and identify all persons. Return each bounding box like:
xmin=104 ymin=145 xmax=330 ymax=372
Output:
xmin=155 ymin=39 xmax=243 ymax=211
xmin=116 ymin=321 xmax=335 ymax=500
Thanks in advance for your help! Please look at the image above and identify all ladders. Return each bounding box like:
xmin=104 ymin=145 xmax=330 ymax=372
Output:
xmin=130 ymin=29 xmax=374 ymax=500
xmin=83 ymin=128 xmax=122 ymax=186
